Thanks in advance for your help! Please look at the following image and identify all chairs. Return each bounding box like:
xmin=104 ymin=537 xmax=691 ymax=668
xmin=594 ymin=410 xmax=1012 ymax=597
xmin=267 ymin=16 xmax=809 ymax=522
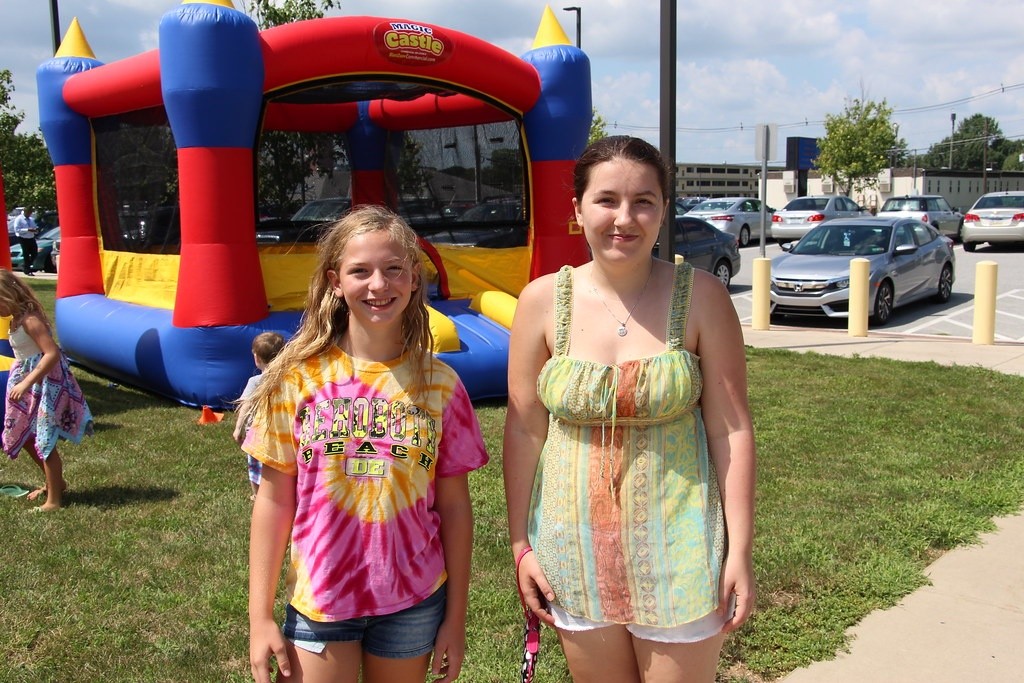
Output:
xmin=824 ymin=230 xmax=892 ymax=250
xmin=897 ymin=200 xmax=906 ymax=210
xmin=989 ymin=198 xmax=1003 ymax=206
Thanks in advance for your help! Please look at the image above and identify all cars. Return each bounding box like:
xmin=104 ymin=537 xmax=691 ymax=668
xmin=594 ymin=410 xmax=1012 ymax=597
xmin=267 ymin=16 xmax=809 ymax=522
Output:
xmin=683 ymin=197 xmax=777 ymax=248
xmin=10 ymin=226 xmax=60 ymax=273
xmin=769 ymin=217 xmax=956 ymax=325
xmin=962 ymin=191 xmax=1024 ymax=251
xmin=6 ymin=206 xmax=49 ymax=234
xmin=255 ymin=197 xmax=478 ymax=245
xmin=771 ymin=196 xmax=874 ymax=248
xmin=652 ymin=216 xmax=741 ymax=289
xmin=876 ymin=195 xmax=965 ymax=244
xmin=674 ymin=198 xmax=707 ymax=215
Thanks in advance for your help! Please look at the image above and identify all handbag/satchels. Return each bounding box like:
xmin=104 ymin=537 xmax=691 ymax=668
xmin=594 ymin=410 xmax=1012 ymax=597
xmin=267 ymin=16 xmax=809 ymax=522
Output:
xmin=516 ymin=547 xmax=540 ymax=683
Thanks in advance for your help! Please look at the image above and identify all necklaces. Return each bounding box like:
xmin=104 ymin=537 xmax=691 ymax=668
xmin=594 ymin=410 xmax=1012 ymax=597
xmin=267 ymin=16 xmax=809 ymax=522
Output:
xmin=589 ymin=256 xmax=654 ymax=337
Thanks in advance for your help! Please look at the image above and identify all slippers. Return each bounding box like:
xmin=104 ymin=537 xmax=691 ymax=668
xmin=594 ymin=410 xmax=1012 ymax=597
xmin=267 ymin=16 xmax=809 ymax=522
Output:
xmin=0 ymin=484 xmax=29 ymax=498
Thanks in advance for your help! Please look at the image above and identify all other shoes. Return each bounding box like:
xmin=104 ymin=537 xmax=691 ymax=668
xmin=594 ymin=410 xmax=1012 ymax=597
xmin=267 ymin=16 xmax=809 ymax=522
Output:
xmin=25 ymin=272 xmax=34 ymax=276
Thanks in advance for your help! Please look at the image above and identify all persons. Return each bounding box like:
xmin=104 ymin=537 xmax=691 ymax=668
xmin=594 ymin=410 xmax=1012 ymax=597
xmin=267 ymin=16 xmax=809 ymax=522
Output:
xmin=13 ymin=205 xmax=39 ymax=277
xmin=233 ymin=333 xmax=285 ymax=501
xmin=502 ymin=134 xmax=757 ymax=683
xmin=0 ymin=268 xmax=96 ymax=511
xmin=222 ymin=203 xmax=489 ymax=683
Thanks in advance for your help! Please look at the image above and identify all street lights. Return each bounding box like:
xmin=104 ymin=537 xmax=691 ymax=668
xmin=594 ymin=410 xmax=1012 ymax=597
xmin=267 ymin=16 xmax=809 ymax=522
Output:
xmin=472 ymin=124 xmax=504 ymax=206
xmin=563 ymin=7 xmax=581 ymax=49
xmin=949 ymin=113 xmax=956 ymax=170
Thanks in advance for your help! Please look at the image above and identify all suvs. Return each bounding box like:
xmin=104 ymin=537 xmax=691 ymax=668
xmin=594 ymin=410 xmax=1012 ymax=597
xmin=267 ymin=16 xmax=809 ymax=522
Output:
xmin=423 ymin=194 xmax=530 ymax=249
xmin=50 ymin=206 xmax=180 ymax=268
